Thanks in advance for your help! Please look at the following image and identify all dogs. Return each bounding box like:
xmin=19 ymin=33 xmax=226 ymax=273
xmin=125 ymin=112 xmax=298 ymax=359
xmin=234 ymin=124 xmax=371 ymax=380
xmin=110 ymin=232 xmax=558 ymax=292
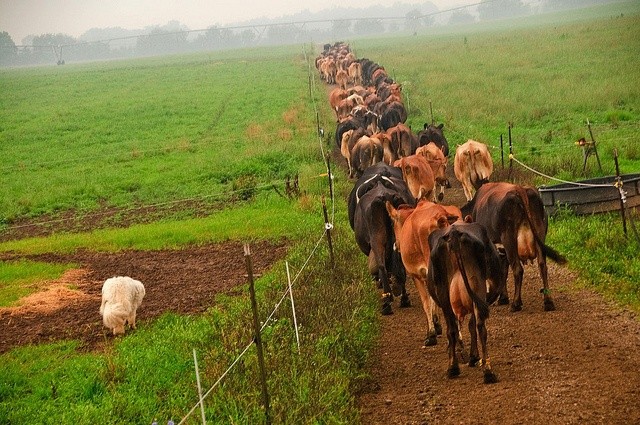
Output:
xmin=99 ymin=275 xmax=145 ymax=336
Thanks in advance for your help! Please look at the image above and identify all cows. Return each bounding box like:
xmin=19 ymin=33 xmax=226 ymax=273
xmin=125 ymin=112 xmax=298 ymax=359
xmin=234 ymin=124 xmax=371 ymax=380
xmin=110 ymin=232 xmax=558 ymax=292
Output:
xmin=394 ymin=153 xmax=437 ymax=203
xmin=350 ymin=135 xmax=384 ymax=178
xmin=314 ymin=41 xmax=407 ymax=129
xmin=414 ymin=142 xmax=452 ymax=201
xmin=460 ymin=182 xmax=568 ymax=312
xmin=454 ymin=138 xmax=495 ymax=202
xmin=417 ymin=122 xmax=449 ymax=156
xmin=385 ymin=199 xmax=463 ymax=346
xmin=370 ymin=130 xmax=398 ymax=166
xmin=348 ymin=127 xmax=371 ymax=153
xmin=341 ymin=129 xmax=354 ymax=177
xmin=425 ymin=215 xmax=505 ymax=384
xmin=335 ymin=121 xmax=359 ymax=149
xmin=353 ymin=181 xmax=415 ymax=315
xmin=348 ymin=161 xmax=408 ymax=233
xmin=385 ymin=121 xmax=418 ymax=158
